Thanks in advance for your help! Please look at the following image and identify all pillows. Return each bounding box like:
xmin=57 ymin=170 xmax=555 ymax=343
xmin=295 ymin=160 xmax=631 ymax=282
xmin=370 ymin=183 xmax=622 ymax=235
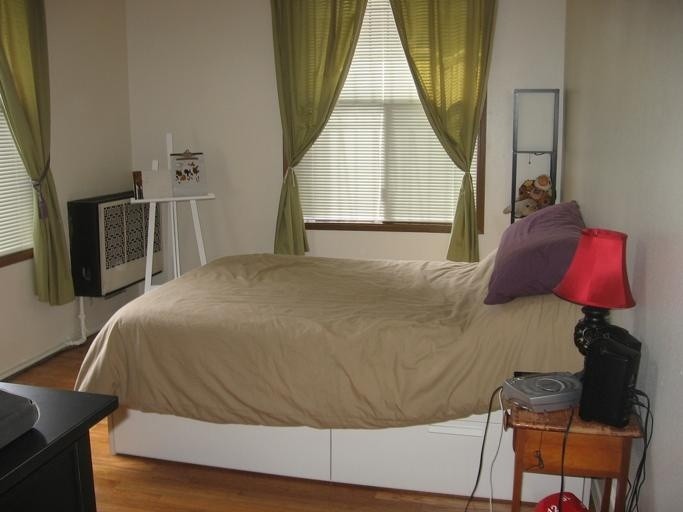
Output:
xmin=483 ymin=199 xmax=590 ymax=307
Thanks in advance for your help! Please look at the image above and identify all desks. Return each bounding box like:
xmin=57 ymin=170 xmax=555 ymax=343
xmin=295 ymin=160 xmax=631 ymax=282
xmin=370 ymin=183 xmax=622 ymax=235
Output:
xmin=504 ymin=363 xmax=644 ymax=510
xmin=0 ymin=377 xmax=118 ymax=512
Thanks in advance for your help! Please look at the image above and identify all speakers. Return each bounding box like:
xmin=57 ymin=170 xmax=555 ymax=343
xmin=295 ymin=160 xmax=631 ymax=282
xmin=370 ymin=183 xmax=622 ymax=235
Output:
xmin=604 ymin=324 xmax=642 ymax=352
xmin=579 ymin=338 xmax=641 ymax=428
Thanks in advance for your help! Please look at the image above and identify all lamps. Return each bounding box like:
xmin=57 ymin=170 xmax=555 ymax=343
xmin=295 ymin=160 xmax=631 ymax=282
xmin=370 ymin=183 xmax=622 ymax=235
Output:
xmin=548 ymin=225 xmax=637 ymax=382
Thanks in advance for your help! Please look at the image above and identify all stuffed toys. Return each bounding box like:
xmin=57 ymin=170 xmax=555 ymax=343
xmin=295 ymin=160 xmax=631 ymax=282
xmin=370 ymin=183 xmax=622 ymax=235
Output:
xmin=503 ymin=175 xmax=552 ymax=217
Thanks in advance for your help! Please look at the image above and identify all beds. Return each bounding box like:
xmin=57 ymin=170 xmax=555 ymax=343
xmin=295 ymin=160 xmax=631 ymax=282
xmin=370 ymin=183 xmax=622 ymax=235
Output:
xmin=102 ymin=249 xmax=600 ymax=510
